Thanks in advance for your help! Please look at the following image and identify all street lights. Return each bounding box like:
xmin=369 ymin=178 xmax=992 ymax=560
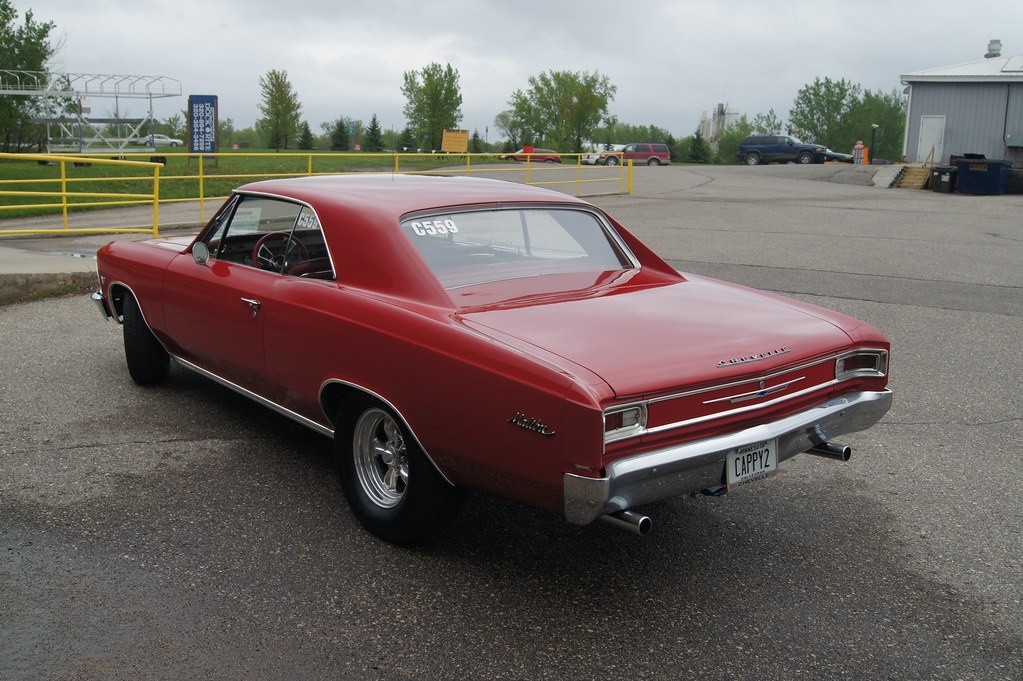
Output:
xmin=870 ymin=124 xmax=879 ymax=164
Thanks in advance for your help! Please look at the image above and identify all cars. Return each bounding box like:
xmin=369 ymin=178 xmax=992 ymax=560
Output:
xmin=599 ymin=144 xmax=670 ymax=166
xmin=581 ymin=148 xmax=631 ymax=167
xmin=92 ymin=176 xmax=894 ymax=551
xmin=499 ymin=148 xmax=561 ymax=164
xmin=137 ymin=134 xmax=183 ymax=147
xmin=779 ymin=148 xmax=855 ymax=165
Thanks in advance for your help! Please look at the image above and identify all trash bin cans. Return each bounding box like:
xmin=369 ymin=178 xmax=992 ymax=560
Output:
xmin=931 ymin=167 xmax=957 ymax=194
xmin=954 ymin=158 xmax=1013 ymax=195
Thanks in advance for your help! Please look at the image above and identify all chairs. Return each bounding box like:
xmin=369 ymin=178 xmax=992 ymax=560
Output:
xmin=287 ymin=257 xmax=333 ymax=280
xmin=434 ymin=244 xmax=495 ymax=269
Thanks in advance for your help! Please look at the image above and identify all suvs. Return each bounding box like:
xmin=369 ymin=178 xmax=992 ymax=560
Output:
xmin=738 ymin=134 xmax=830 ymax=165
